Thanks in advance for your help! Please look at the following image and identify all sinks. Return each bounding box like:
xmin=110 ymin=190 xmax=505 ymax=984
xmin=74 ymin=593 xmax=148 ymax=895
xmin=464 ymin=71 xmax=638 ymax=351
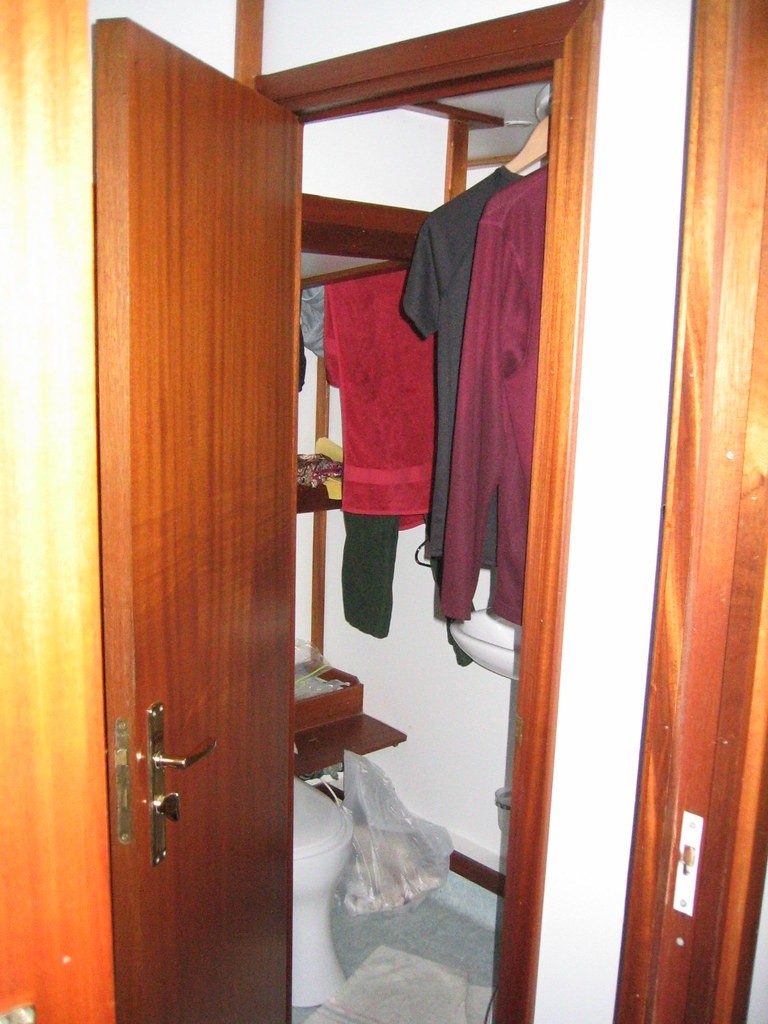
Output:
xmin=449 ymin=608 xmax=522 ymax=685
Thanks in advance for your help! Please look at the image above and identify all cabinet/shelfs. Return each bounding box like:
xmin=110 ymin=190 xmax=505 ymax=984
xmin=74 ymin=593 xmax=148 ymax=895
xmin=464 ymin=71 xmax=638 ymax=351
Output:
xmin=296 ymin=455 xmax=406 ymax=781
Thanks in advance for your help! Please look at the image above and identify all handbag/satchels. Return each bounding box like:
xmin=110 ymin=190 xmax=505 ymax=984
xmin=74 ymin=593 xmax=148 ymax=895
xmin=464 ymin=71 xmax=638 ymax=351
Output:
xmin=322 ymin=748 xmax=453 ymax=916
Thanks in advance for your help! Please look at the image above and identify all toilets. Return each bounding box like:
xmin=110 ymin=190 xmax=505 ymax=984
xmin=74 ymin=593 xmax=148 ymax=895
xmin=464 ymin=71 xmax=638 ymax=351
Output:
xmin=292 ymin=773 xmax=356 ymax=1010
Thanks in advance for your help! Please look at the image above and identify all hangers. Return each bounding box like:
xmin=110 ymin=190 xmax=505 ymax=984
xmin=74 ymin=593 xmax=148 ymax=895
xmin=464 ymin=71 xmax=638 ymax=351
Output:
xmin=505 ymin=116 xmax=549 ymax=172
